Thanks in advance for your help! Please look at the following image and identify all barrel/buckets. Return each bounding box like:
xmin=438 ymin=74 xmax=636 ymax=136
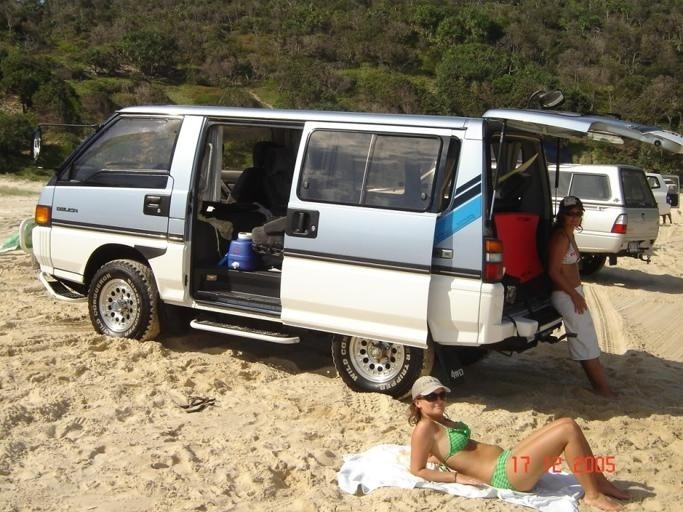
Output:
xmin=227 ymin=231 xmax=259 ymax=272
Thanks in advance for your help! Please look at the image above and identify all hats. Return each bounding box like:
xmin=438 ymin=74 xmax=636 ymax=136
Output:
xmin=411 ymin=376 xmax=451 ymax=400
xmin=560 ymin=196 xmax=583 ymax=209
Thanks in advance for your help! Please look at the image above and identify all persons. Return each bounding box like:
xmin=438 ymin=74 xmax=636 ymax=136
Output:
xmin=662 ymin=193 xmax=672 ymax=225
xmin=544 ymin=195 xmax=616 ymax=397
xmin=407 ymin=375 xmax=633 ymax=511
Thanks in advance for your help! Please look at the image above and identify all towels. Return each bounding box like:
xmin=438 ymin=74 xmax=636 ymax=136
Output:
xmin=336 ymin=443 xmax=585 ymax=512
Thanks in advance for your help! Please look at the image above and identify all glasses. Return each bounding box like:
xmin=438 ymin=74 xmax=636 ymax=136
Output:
xmin=567 ymin=211 xmax=582 ymax=217
xmin=418 ymin=392 xmax=446 ymax=401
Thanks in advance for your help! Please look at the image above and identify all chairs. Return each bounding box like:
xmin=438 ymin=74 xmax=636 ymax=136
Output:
xmin=200 ymin=167 xmax=288 ymax=237
